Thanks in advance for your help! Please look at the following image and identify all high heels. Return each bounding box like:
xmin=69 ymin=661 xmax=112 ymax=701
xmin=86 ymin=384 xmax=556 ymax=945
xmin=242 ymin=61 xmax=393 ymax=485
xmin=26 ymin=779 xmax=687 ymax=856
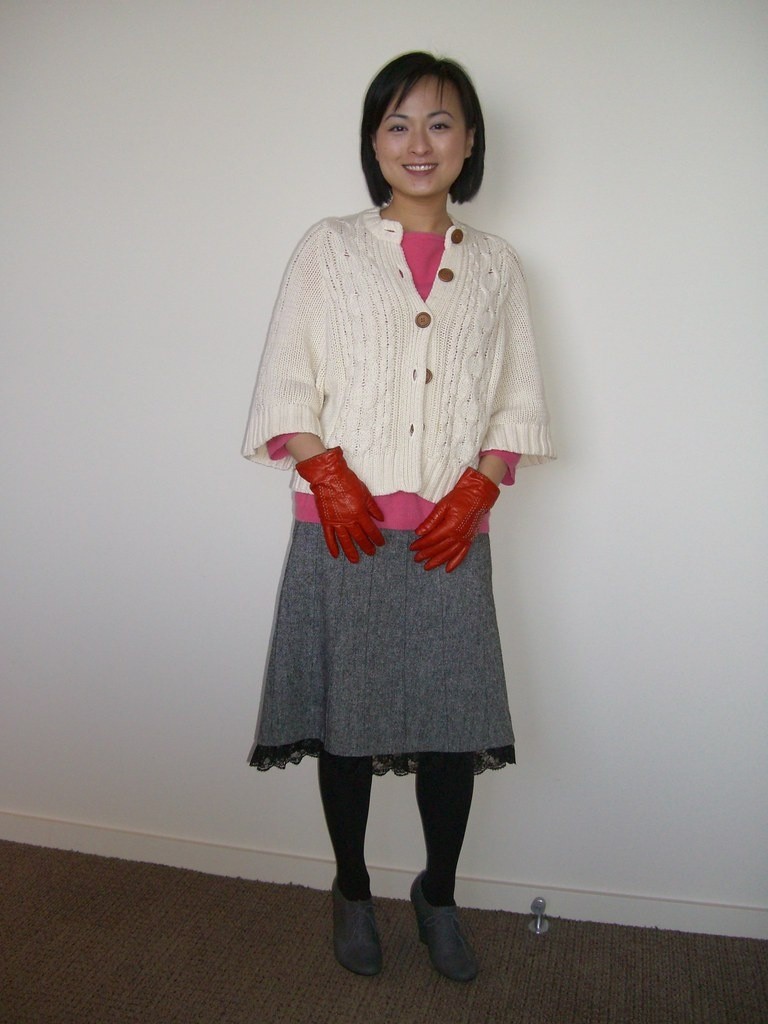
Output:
xmin=331 ymin=876 xmax=382 ymax=975
xmin=410 ymin=871 xmax=477 ymax=981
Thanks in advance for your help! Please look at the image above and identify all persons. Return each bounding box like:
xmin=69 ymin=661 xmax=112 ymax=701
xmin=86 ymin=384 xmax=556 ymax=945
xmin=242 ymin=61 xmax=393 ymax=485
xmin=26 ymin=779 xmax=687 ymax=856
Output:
xmin=245 ymin=53 xmax=557 ymax=983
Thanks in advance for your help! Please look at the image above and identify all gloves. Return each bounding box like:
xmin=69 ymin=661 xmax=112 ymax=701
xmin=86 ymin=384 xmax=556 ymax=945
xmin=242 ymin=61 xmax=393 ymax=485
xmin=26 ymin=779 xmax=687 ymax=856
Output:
xmin=296 ymin=447 xmax=386 ymax=563
xmin=409 ymin=466 xmax=500 ymax=574
